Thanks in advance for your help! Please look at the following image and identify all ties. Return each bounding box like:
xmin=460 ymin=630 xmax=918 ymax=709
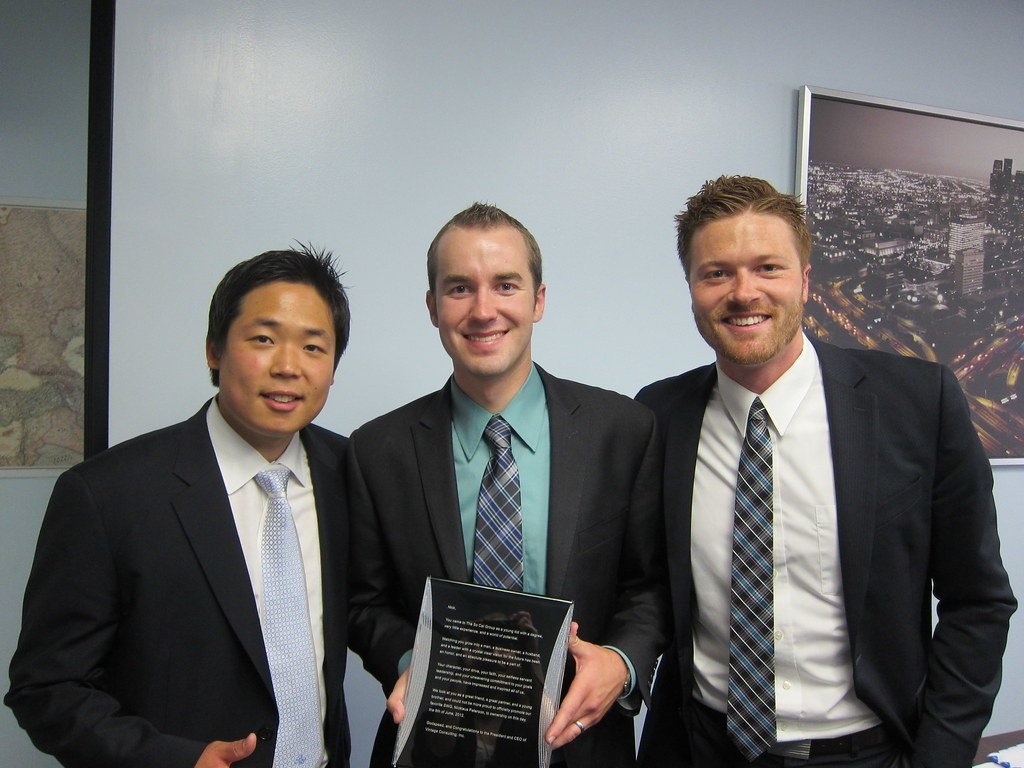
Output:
xmin=472 ymin=414 xmax=524 ymax=592
xmin=251 ymin=464 xmax=323 ymax=768
xmin=726 ymin=397 xmax=776 ymax=762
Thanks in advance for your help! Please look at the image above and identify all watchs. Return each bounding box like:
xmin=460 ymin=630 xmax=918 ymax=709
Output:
xmin=624 ymin=668 xmax=631 ymax=694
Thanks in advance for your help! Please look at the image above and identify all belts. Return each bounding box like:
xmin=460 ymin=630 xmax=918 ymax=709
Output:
xmin=765 ymin=720 xmax=887 ymax=760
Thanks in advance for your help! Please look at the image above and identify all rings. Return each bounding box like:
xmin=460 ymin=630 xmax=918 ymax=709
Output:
xmin=576 ymin=720 xmax=585 ymax=734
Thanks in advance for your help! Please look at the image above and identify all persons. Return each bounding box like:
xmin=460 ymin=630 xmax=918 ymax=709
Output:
xmin=345 ymin=198 xmax=659 ymax=768
xmin=634 ymin=172 xmax=1022 ymax=768
xmin=2 ymin=236 xmax=415 ymax=768
